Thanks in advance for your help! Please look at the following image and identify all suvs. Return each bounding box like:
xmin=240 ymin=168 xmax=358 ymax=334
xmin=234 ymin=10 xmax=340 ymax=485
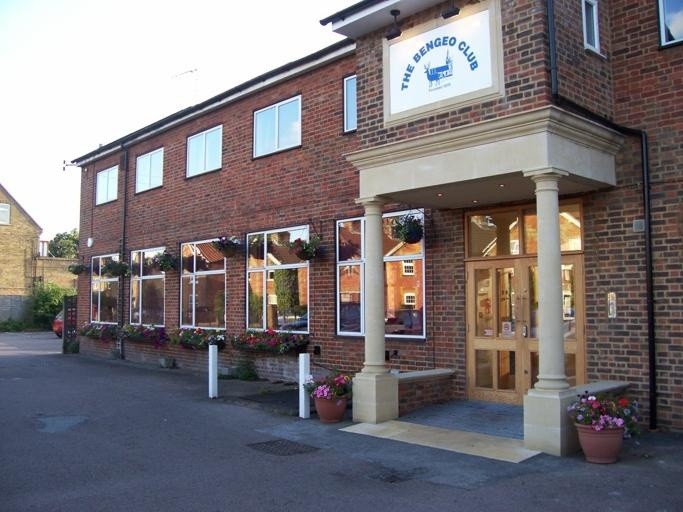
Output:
xmin=279 ymin=299 xmax=361 ymax=332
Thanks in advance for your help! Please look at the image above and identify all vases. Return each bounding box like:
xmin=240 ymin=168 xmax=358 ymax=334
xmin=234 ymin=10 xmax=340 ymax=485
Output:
xmin=574 ymin=419 xmax=628 ymax=464
xmin=315 ymin=397 xmax=347 ymax=423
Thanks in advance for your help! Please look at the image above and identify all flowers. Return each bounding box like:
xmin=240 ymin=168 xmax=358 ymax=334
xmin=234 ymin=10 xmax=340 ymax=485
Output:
xmin=177 ymin=324 xmax=308 ymax=356
xmin=80 ymin=321 xmax=168 ymax=345
xmin=282 ymin=235 xmax=322 ymax=259
xmin=152 ymin=252 xmax=177 ymax=269
xmin=303 ymin=367 xmax=352 ymax=400
xmin=566 ymin=388 xmax=643 ymax=438
xmin=208 ymin=235 xmax=239 ymax=253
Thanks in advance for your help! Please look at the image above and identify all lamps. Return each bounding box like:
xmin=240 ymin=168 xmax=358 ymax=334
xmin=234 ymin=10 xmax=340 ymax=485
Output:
xmin=442 ymin=0 xmax=460 ymax=18
xmin=386 ymin=9 xmax=403 ymax=40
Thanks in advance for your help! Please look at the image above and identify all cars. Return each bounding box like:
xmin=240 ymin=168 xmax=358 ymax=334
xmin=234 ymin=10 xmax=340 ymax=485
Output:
xmin=53 ymin=308 xmax=77 ymax=337
xmin=384 ymin=309 xmax=423 ymax=336
